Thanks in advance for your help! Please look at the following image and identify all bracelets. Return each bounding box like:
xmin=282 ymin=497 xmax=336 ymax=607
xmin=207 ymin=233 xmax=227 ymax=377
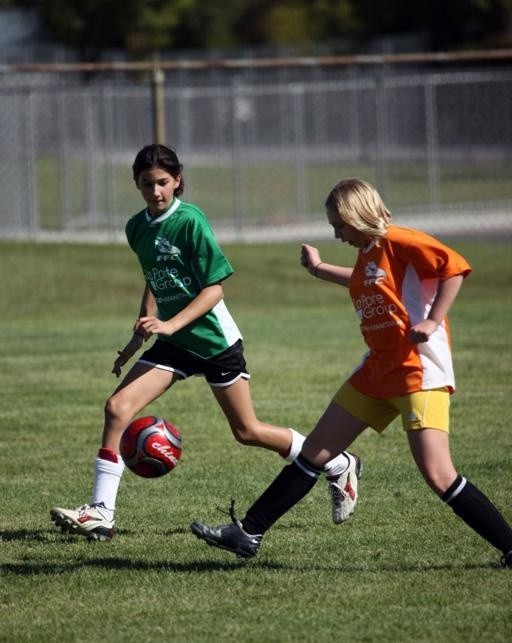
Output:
xmin=134 ymin=333 xmax=145 ymax=339
xmin=315 ymin=262 xmax=323 ymax=277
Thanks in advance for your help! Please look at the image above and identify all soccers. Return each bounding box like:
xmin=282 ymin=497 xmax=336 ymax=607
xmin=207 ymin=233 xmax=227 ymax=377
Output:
xmin=119 ymin=416 xmax=182 ymax=478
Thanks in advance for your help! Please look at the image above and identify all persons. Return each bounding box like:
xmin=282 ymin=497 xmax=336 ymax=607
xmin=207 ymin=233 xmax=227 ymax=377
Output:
xmin=49 ymin=141 xmax=363 ymax=542
xmin=189 ymin=180 xmax=512 ymax=569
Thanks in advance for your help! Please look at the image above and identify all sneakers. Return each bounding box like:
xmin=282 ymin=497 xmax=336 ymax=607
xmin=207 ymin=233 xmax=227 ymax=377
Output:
xmin=324 ymin=450 xmax=363 ymax=525
xmin=49 ymin=501 xmax=116 ymax=542
xmin=190 ymin=496 xmax=264 ymax=559
